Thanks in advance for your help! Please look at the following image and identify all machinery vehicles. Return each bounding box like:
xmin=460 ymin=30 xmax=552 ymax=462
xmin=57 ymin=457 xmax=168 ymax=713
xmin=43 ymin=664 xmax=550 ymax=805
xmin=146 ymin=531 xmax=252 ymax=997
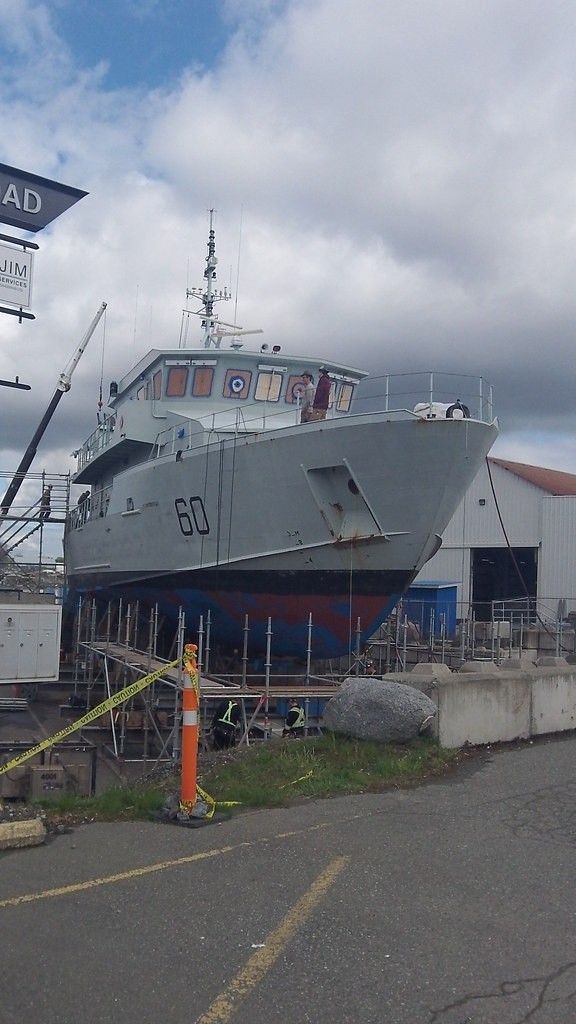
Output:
xmin=0 ymin=300 xmax=109 ymax=527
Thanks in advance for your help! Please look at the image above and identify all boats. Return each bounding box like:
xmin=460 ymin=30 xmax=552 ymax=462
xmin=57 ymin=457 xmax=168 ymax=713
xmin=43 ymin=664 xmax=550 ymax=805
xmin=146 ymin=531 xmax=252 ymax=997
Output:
xmin=57 ymin=208 xmax=504 ymax=720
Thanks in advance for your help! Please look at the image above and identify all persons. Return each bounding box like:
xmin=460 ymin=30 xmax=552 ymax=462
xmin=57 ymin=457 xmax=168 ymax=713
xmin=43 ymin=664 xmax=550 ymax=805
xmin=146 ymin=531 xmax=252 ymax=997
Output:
xmin=39 ymin=488 xmax=51 ymax=519
xmin=300 ymin=370 xmax=316 ymax=423
xmin=212 ymin=698 xmax=242 ymax=751
xmin=314 ymin=365 xmax=331 ymax=422
xmin=282 ymin=697 xmax=306 ymax=739
xmin=77 ymin=490 xmax=90 ymax=521
xmin=366 ymin=659 xmax=377 ymax=675
